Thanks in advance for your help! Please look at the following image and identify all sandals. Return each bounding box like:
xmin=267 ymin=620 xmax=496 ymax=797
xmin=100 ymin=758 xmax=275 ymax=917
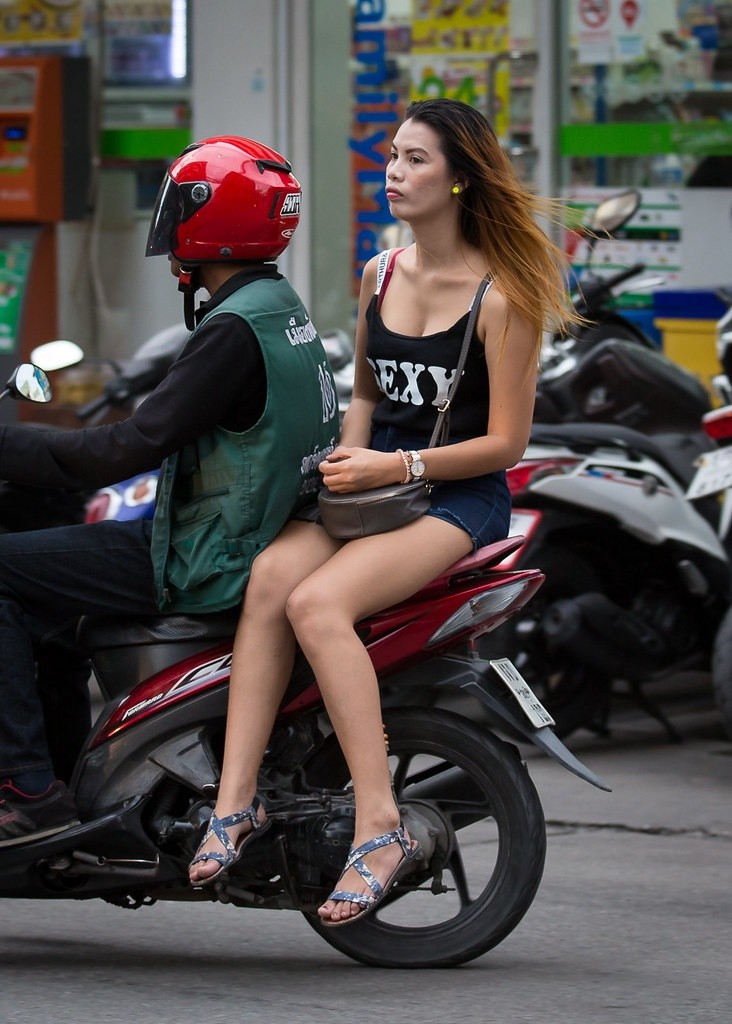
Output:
xmin=317 ymin=821 xmax=418 ymax=926
xmin=188 ymin=795 xmax=272 ymax=887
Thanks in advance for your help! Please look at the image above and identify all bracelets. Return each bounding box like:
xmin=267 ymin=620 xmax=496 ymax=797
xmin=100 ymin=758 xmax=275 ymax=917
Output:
xmin=397 ymin=447 xmax=411 ymax=486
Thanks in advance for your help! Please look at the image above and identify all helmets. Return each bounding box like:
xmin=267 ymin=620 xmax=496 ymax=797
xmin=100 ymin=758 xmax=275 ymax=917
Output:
xmin=145 ymin=135 xmax=302 ymax=266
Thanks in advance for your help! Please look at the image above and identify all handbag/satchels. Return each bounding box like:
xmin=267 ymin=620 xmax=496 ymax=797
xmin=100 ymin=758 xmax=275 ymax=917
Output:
xmin=317 ymin=479 xmax=435 ymax=540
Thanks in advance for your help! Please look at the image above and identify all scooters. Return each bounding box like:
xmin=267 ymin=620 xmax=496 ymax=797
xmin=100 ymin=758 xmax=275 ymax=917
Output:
xmin=76 ymin=182 xmax=732 ymax=746
xmin=0 ymin=340 xmax=616 ymax=970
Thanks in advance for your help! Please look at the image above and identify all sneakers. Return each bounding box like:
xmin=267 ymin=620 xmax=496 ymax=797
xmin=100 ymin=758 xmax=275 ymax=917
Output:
xmin=0 ymin=781 xmax=83 ymax=847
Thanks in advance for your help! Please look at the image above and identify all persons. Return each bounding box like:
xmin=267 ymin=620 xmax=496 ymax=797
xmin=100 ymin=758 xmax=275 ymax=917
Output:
xmin=191 ymin=97 xmax=544 ymax=927
xmin=0 ymin=134 xmax=342 ymax=847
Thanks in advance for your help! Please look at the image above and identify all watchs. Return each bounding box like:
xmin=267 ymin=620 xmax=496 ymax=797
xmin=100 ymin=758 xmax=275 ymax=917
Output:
xmin=407 ymin=450 xmax=424 ymax=483
